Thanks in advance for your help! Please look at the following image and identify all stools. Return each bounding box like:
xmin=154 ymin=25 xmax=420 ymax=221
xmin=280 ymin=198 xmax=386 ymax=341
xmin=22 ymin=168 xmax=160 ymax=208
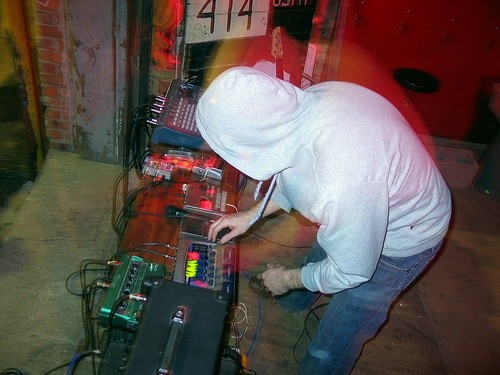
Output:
xmin=393 ymin=68 xmax=440 ymax=101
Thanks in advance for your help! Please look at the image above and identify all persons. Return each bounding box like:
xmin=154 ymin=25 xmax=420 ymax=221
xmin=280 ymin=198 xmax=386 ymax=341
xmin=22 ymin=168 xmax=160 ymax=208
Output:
xmin=195 ymin=66 xmax=452 ymax=375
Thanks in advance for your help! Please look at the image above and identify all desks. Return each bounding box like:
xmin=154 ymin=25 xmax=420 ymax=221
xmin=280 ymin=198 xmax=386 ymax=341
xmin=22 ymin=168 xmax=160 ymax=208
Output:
xmin=118 ymin=146 xmax=239 ymax=273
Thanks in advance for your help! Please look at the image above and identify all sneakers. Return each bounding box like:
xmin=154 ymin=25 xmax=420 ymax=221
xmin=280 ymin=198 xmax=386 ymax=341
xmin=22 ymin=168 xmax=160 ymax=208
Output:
xmin=249 ymin=277 xmax=299 ymax=318
